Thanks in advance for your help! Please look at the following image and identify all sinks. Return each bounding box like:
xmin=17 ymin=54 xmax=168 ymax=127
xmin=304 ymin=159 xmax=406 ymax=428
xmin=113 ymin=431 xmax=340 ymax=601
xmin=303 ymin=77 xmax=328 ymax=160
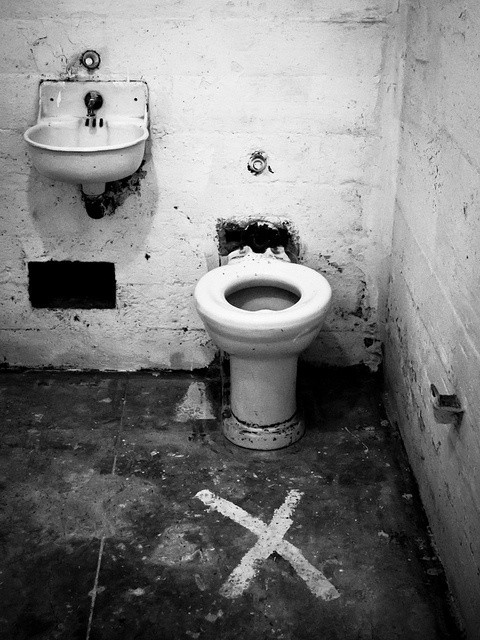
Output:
xmin=23 ymin=117 xmax=150 ymax=183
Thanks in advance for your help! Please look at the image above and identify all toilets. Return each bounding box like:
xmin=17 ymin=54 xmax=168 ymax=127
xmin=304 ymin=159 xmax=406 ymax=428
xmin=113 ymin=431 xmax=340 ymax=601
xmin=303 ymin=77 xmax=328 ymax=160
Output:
xmin=194 ymin=246 xmax=332 ymax=451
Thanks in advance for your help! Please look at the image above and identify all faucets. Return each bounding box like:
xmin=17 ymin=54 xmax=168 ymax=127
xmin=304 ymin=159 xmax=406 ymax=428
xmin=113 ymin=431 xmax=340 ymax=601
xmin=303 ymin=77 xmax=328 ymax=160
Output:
xmin=78 ymin=49 xmax=102 ymax=76
xmin=83 ymin=90 xmax=103 ymax=118
